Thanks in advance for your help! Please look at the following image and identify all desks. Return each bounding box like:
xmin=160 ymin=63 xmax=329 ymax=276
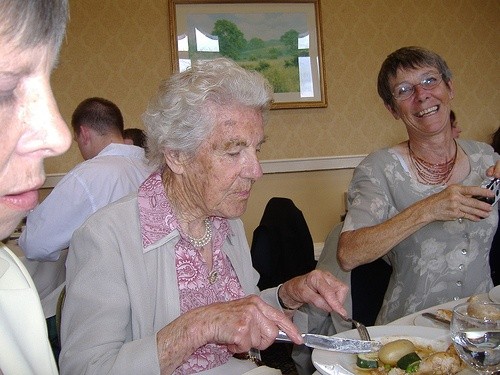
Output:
xmin=4 ymin=221 xmax=68 ymax=319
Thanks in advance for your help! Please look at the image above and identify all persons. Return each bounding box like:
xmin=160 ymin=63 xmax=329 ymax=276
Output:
xmin=59 ymin=56 xmax=351 ymax=375
xmin=450 ymin=110 xmax=463 ymax=139
xmin=337 ymin=46 xmax=499 ymax=325
xmin=0 ymin=0 xmax=73 ymax=375
xmin=122 ymin=128 xmax=147 ymax=148
xmin=18 ymin=98 xmax=158 ymax=261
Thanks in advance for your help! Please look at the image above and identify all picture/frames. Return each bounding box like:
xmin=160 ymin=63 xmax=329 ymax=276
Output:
xmin=167 ymin=0 xmax=329 ymax=110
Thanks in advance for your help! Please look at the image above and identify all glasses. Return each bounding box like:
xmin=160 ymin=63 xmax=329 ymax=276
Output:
xmin=390 ymin=72 xmax=443 ymax=101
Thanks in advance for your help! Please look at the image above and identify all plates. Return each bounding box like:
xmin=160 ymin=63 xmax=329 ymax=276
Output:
xmin=311 ymin=324 xmax=480 ymax=375
xmin=414 ymin=314 xmax=452 ymax=330
xmin=448 ymin=296 xmax=500 ymax=328
xmin=487 ymin=285 xmax=500 ymax=304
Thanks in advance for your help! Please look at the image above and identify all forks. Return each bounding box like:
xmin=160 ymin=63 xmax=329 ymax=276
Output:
xmin=336 ymin=312 xmax=372 ymax=341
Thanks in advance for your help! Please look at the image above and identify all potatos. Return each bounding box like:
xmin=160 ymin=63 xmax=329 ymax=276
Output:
xmin=378 ymin=339 xmax=415 ymax=366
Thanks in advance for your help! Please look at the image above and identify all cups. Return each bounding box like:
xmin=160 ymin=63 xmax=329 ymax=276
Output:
xmin=448 ymin=301 xmax=500 ymax=375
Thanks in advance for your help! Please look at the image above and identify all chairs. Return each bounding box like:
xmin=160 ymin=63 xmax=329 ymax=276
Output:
xmin=251 ymin=197 xmax=318 ymax=375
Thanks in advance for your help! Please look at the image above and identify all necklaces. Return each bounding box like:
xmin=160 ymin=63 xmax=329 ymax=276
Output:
xmin=184 ymin=218 xmax=212 ymax=247
xmin=406 ymin=138 xmax=457 ymax=188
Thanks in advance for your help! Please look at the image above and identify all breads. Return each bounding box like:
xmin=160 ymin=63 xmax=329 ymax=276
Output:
xmin=466 ymin=295 xmax=500 ymax=321
xmin=435 ymin=309 xmax=453 ymax=321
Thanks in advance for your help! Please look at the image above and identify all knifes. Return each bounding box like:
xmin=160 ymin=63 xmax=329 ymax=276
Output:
xmin=276 ymin=329 xmax=384 ymax=354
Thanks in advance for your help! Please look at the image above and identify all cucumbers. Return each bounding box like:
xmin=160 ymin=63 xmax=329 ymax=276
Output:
xmin=356 ymin=354 xmax=379 ymax=368
xmin=397 ymin=352 xmax=422 ymax=373
